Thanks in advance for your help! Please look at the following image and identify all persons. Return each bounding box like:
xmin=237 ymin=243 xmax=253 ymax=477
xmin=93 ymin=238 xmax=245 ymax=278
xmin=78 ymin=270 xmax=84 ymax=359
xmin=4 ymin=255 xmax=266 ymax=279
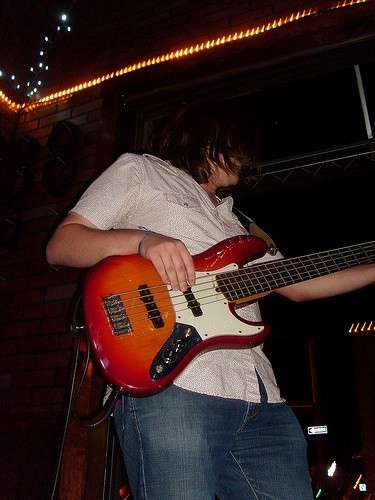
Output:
xmin=44 ymin=102 xmax=374 ymax=500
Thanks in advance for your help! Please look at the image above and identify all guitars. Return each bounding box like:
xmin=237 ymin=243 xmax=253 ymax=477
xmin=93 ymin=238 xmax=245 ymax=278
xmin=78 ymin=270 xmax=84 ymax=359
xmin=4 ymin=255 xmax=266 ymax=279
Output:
xmin=81 ymin=233 xmax=375 ymax=398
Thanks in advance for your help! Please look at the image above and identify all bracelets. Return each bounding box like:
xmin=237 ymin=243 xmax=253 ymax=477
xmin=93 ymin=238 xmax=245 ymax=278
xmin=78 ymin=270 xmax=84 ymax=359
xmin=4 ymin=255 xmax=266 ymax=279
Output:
xmin=137 ymin=228 xmax=153 ymax=255
